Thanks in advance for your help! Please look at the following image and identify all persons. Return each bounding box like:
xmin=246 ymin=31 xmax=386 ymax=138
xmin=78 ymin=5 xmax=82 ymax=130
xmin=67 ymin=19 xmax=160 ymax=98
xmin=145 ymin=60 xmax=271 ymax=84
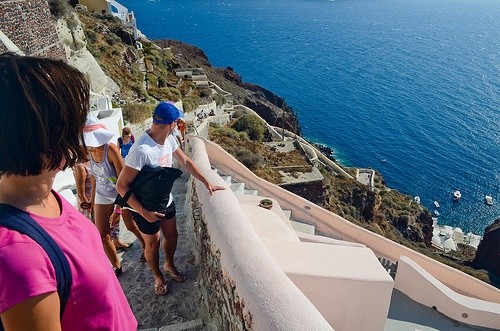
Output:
xmin=75 ymin=103 xmax=226 ymax=295
xmin=0 ymin=52 xmax=138 ymax=331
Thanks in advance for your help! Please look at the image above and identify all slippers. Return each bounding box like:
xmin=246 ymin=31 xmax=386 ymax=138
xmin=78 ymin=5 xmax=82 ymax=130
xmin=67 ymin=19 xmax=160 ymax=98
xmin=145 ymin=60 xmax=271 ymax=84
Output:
xmin=153 ymin=283 xmax=168 ymax=296
xmin=166 ymin=271 xmax=187 ymax=283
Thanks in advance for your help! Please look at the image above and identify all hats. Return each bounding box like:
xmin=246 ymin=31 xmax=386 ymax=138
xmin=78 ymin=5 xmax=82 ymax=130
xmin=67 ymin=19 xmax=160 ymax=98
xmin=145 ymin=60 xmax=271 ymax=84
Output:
xmin=153 ymin=102 xmax=184 ymax=124
xmin=79 ymin=112 xmax=114 ymax=148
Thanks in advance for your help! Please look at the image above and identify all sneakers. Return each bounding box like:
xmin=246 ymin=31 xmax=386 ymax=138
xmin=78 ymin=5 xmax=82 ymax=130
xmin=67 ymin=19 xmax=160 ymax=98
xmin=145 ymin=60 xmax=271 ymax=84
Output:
xmin=139 ymin=253 xmax=147 ymax=262
xmin=115 ymin=266 xmax=122 ymax=276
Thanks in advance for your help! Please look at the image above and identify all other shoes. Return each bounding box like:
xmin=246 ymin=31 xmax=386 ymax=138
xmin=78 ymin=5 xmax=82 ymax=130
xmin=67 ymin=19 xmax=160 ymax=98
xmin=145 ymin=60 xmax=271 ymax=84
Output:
xmin=116 ymin=243 xmax=134 ymax=250
